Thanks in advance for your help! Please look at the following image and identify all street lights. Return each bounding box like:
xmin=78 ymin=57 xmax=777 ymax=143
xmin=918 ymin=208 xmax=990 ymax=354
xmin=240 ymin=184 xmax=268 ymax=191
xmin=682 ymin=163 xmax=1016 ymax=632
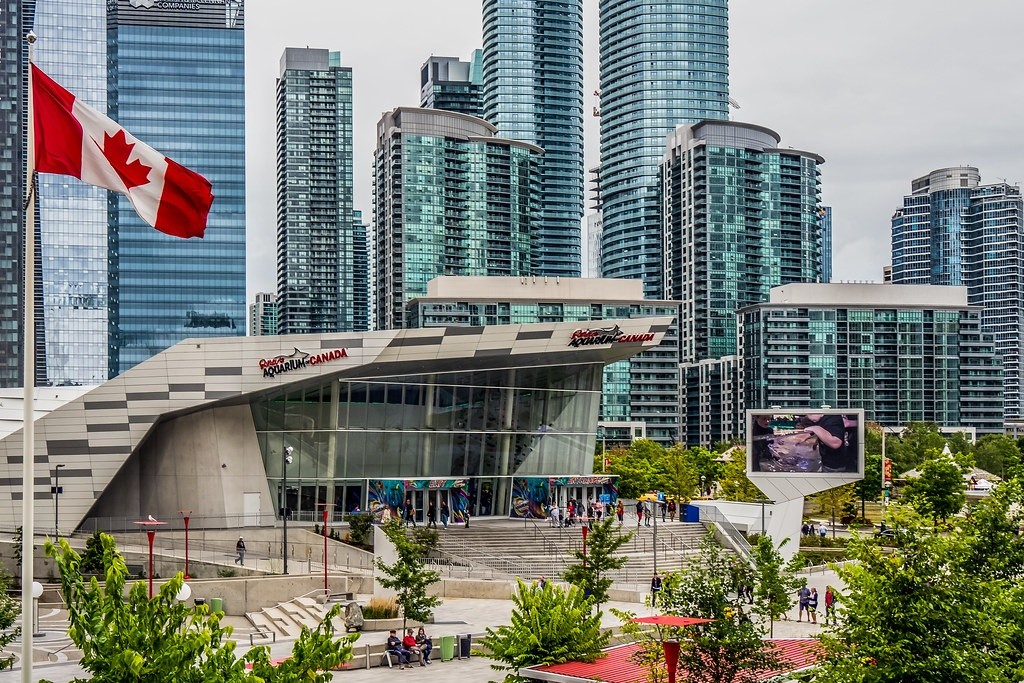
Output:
xmin=751 ymin=499 xmax=776 ymax=536
xmin=644 ymin=499 xmax=665 ymax=574
xmin=177 ymin=510 xmax=195 ymax=579
xmin=56 ymin=464 xmax=66 ymax=542
xmin=315 ymin=503 xmax=338 ymax=603
xmin=571 ymin=517 xmax=596 ymax=619
xmin=132 ymin=522 xmax=168 ymax=600
xmin=630 ymin=617 xmax=718 ymax=683
xmin=282 ymin=446 xmax=293 ymax=575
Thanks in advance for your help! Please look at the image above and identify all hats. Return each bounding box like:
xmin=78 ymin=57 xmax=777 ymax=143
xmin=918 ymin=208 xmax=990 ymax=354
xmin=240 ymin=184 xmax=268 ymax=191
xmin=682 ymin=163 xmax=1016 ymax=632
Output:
xmin=801 ymin=582 xmax=807 ymax=585
xmin=239 ymin=536 xmax=243 ymax=538
xmin=408 ymin=628 xmax=414 ymax=633
xmin=804 ymin=521 xmax=807 ymax=525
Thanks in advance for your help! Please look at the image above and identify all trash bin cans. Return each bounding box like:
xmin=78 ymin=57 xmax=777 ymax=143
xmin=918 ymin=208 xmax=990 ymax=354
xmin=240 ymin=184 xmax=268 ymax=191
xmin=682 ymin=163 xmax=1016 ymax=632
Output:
xmin=194 ymin=598 xmax=206 ymax=615
xmin=211 ymin=598 xmax=222 ymax=613
xmin=456 ymin=634 xmax=472 ymax=660
xmin=439 ymin=635 xmax=455 ymax=662
xmin=680 ymin=504 xmax=699 ymax=522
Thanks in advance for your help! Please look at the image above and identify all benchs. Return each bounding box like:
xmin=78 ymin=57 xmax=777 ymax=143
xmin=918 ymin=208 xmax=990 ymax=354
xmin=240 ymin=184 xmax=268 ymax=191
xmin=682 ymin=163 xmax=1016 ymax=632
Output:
xmin=316 ymin=592 xmax=358 ymax=604
xmin=381 ymin=640 xmax=433 ymax=667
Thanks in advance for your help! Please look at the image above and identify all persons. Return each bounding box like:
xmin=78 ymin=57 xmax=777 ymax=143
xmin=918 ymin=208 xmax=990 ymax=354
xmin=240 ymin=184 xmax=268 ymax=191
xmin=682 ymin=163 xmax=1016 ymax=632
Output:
xmin=416 ymin=627 xmax=432 ymax=664
xmin=651 ymin=572 xmax=673 ymax=607
xmin=804 ymin=412 xmax=847 ymax=472
xmin=388 ymin=630 xmax=414 ymax=669
xmin=549 ymin=493 xmax=676 ymax=530
xmin=537 ymin=575 xmax=546 ymax=588
xmin=402 ymin=628 xmax=428 ymax=666
xmin=752 ymin=414 xmax=788 ymax=471
xmin=736 ymin=569 xmax=838 ymax=626
xmin=801 ymin=521 xmax=827 ymax=537
xmin=880 ymin=521 xmax=886 ymax=531
xmin=352 ymin=499 xmax=470 ymax=529
xmin=235 ymin=537 xmax=246 ymax=566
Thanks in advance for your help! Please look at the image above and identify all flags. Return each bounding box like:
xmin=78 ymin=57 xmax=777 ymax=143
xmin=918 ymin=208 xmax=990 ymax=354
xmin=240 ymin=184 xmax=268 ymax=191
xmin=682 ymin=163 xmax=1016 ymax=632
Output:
xmin=30 ymin=61 xmax=215 ymax=238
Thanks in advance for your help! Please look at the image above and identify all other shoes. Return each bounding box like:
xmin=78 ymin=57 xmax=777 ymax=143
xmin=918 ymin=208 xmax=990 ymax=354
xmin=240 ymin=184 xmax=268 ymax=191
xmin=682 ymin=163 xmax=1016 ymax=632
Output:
xmin=796 ymin=620 xmax=801 ymax=622
xmin=414 ymin=650 xmax=420 ymax=654
xmin=811 ymin=621 xmax=817 ymax=624
xmin=407 ymin=664 xmax=412 ymax=667
xmin=235 ymin=559 xmax=238 ymax=565
xmin=399 ymin=666 xmax=404 ymax=670
xmin=419 ymin=662 xmax=425 ymax=666
xmin=427 ymin=660 xmax=432 ymax=664
xmin=423 ymin=659 xmax=425 ymax=665
xmin=241 ymin=563 xmax=244 ymax=565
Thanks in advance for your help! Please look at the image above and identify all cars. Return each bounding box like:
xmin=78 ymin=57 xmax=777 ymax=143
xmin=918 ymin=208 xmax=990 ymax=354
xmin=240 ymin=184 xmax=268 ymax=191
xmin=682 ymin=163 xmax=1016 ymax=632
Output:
xmin=875 ymin=530 xmax=896 ymax=539
